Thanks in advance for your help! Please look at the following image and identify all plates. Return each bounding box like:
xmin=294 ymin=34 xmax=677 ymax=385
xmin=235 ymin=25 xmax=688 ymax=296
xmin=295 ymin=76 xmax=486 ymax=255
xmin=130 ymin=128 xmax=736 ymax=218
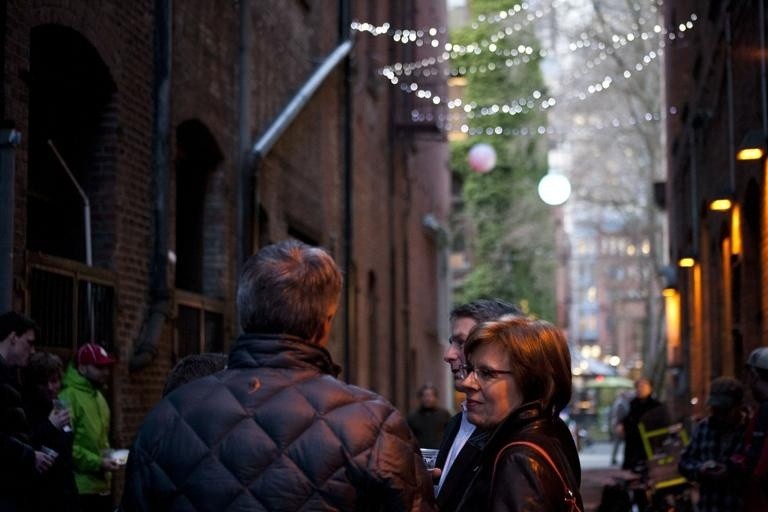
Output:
xmin=109 ymin=448 xmax=130 ymax=466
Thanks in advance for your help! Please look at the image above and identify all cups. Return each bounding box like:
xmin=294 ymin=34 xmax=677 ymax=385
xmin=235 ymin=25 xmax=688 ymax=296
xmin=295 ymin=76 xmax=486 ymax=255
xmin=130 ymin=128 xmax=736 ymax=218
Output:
xmin=43 ymin=447 xmax=59 ymax=467
xmin=52 ymin=398 xmax=68 ymax=413
xmin=418 ymin=447 xmax=440 ymax=477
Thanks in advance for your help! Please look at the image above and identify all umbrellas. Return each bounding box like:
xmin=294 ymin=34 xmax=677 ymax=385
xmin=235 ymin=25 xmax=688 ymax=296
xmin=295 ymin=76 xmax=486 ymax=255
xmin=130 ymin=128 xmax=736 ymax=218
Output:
xmin=569 ymin=348 xmax=612 ymax=376
xmin=579 ymin=376 xmax=634 ymax=404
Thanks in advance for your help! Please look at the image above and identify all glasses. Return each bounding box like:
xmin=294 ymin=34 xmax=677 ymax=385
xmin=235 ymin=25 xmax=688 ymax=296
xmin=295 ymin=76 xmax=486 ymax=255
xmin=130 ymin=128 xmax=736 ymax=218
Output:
xmin=462 ymin=365 xmax=514 ymax=382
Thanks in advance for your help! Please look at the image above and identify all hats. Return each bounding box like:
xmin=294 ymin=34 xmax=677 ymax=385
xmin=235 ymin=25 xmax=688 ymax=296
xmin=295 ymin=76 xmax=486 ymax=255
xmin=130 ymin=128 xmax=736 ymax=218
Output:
xmin=706 ymin=375 xmax=744 ymax=407
xmin=78 ymin=343 xmax=118 ymax=366
xmin=740 ymin=347 xmax=768 ymax=369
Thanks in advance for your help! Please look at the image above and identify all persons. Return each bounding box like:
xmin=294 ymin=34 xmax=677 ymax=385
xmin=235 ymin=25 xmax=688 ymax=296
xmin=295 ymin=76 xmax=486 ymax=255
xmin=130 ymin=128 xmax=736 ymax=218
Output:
xmin=0 ymin=310 xmax=56 ymax=510
xmin=29 ymin=351 xmax=79 ymax=510
xmin=117 ymin=235 xmax=440 ymax=511
xmin=606 ymin=346 xmax=768 ymax=512
xmin=455 ymin=312 xmax=584 ymax=511
xmin=58 ymin=342 xmax=120 ymax=511
xmin=163 ymin=351 xmax=229 ymax=398
xmin=404 ymin=381 xmax=454 ymax=486
xmin=427 ymin=293 xmax=525 ymax=512
